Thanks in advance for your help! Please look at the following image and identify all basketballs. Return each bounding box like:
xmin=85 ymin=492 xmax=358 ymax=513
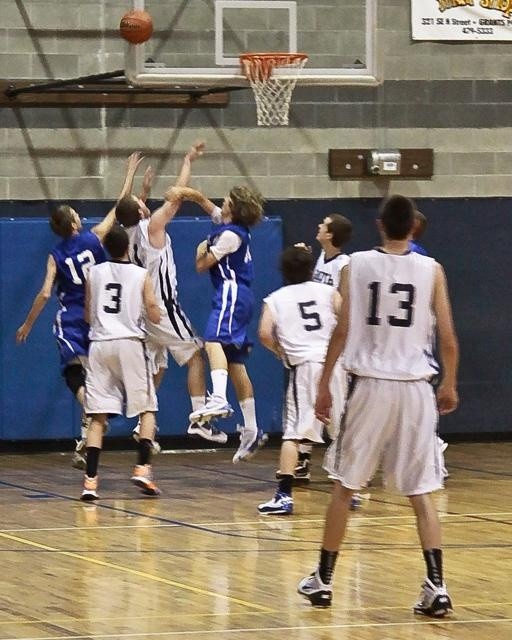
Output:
xmin=120 ymin=10 xmax=153 ymax=44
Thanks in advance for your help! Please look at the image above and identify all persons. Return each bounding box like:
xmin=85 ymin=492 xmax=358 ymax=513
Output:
xmin=117 ymin=139 xmax=228 ymax=455
xmin=79 ymin=225 xmax=164 ymax=499
xmin=274 ymin=214 xmax=354 ymax=481
xmin=16 ymin=151 xmax=147 ymax=470
xmin=163 ymin=184 xmax=271 ymax=466
xmin=256 ymin=247 xmax=355 ymax=515
xmin=409 ymin=210 xmax=450 ymax=481
xmin=298 ymin=194 xmax=461 ymax=617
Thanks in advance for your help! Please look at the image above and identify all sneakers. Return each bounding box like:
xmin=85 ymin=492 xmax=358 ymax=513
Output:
xmin=71 ymin=390 xmax=268 ymax=498
xmin=258 ymin=434 xmax=454 ymax=616
